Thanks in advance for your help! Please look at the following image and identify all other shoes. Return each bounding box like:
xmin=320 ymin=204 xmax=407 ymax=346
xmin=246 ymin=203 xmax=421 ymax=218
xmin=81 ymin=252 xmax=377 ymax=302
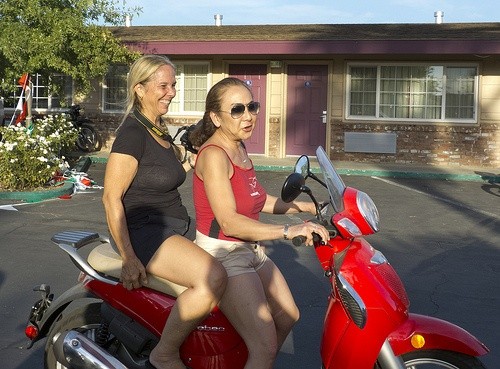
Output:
xmin=147 ymin=351 xmax=189 ymax=369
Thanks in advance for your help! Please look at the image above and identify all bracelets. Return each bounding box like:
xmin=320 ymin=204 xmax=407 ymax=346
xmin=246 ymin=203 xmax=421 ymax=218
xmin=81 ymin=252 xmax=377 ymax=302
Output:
xmin=283 ymin=223 xmax=289 ymax=239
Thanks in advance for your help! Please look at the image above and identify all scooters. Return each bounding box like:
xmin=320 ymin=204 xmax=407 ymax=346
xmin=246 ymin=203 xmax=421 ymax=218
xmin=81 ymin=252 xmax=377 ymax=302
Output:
xmin=33 ymin=104 xmax=98 ymax=152
xmin=25 ymin=145 xmax=490 ymax=369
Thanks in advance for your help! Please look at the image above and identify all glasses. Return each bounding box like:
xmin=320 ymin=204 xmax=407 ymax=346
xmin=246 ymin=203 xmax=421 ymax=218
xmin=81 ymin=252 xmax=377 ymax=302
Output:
xmin=219 ymin=101 xmax=261 ymax=119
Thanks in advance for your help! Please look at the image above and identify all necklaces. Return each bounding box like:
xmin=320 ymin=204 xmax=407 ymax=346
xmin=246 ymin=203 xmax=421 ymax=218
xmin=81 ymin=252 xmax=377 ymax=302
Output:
xmin=234 ymin=144 xmax=247 ymax=162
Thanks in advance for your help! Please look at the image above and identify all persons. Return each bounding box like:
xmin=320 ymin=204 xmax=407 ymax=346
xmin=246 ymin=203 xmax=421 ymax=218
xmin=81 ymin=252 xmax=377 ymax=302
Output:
xmin=102 ymin=55 xmax=228 ymax=369
xmin=190 ymin=75 xmax=330 ymax=369
